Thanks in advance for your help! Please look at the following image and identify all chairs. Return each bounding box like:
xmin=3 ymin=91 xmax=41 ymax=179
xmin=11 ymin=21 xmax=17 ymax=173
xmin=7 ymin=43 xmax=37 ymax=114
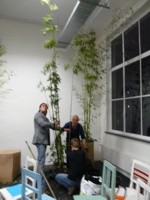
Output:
xmin=73 ymin=160 xmax=116 ymax=200
xmin=108 ymin=159 xmax=150 ymax=200
xmin=0 ymin=156 xmax=38 ymax=200
xmin=21 ymin=168 xmax=57 ymax=200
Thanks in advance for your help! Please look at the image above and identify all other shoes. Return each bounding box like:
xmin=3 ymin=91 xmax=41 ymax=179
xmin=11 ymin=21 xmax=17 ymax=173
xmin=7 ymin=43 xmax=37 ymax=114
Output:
xmin=68 ymin=186 xmax=74 ymax=195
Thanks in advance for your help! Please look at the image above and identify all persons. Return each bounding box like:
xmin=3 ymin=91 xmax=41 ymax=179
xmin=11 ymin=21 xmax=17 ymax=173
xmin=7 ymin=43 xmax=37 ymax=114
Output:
xmin=54 ymin=138 xmax=89 ymax=196
xmin=57 ymin=115 xmax=89 ymax=173
xmin=32 ymin=103 xmax=71 ymax=194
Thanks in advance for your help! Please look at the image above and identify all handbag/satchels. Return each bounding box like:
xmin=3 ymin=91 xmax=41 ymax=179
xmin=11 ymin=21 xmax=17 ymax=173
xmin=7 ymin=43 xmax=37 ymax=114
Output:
xmin=80 ymin=175 xmax=103 ymax=196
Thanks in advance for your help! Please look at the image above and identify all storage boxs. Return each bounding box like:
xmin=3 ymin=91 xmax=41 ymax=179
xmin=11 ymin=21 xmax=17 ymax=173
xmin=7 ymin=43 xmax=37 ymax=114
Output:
xmin=0 ymin=148 xmax=21 ymax=183
